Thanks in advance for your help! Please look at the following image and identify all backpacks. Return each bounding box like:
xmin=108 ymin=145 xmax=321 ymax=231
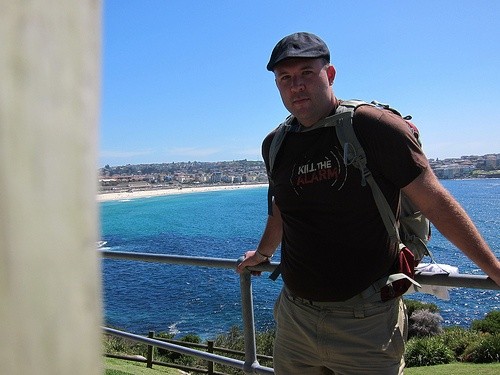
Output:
xmin=268 ymin=98 xmax=447 ymax=292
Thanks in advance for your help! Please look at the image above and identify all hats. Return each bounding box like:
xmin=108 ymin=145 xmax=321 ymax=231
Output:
xmin=266 ymin=32 xmax=330 ymax=72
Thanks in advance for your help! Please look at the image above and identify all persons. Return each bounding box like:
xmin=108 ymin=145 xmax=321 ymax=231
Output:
xmin=234 ymin=32 xmax=500 ymax=375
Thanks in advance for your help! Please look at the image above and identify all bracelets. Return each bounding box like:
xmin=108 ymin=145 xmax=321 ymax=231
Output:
xmin=254 ymin=251 xmax=274 ymax=263
